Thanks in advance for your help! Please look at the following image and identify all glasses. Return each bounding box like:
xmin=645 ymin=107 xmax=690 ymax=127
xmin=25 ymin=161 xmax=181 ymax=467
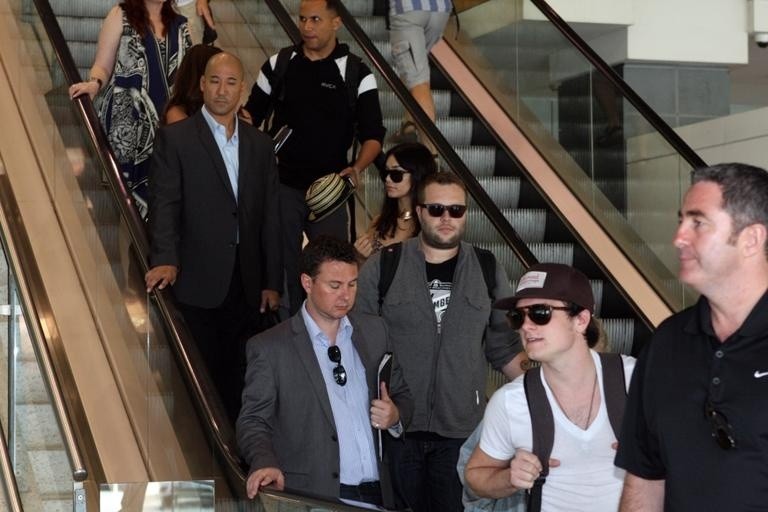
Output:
xmin=420 ymin=202 xmax=467 ymax=218
xmin=506 ymin=306 xmax=576 ymax=328
xmin=704 ymin=398 xmax=736 ymax=451
xmin=327 ymin=345 xmax=347 ymax=386
xmin=380 ymin=169 xmax=411 ymax=181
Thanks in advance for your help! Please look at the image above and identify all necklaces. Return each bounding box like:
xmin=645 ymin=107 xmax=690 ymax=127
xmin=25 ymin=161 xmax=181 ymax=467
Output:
xmin=544 ymin=372 xmax=599 ymax=431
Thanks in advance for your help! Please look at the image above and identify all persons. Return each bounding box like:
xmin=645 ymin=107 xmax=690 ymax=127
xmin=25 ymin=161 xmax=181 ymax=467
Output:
xmin=610 ymin=159 xmax=768 ymax=511
xmin=232 ymin=233 xmax=418 ymax=512
xmin=69 ymin=1 xmax=222 ymax=306
xmin=388 ymin=1 xmax=454 ymax=168
xmin=464 ymin=258 xmax=646 ymax=512
xmin=148 ymin=52 xmax=285 ymax=427
xmin=236 ymin=2 xmax=385 ymax=233
xmin=350 ymin=142 xmax=439 ymax=270
xmin=351 ymin=171 xmax=536 ymax=512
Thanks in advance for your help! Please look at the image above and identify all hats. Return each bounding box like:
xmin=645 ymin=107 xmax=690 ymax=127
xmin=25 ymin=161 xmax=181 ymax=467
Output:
xmin=305 ymin=172 xmax=358 ymax=221
xmin=493 ymin=262 xmax=594 ymax=312
